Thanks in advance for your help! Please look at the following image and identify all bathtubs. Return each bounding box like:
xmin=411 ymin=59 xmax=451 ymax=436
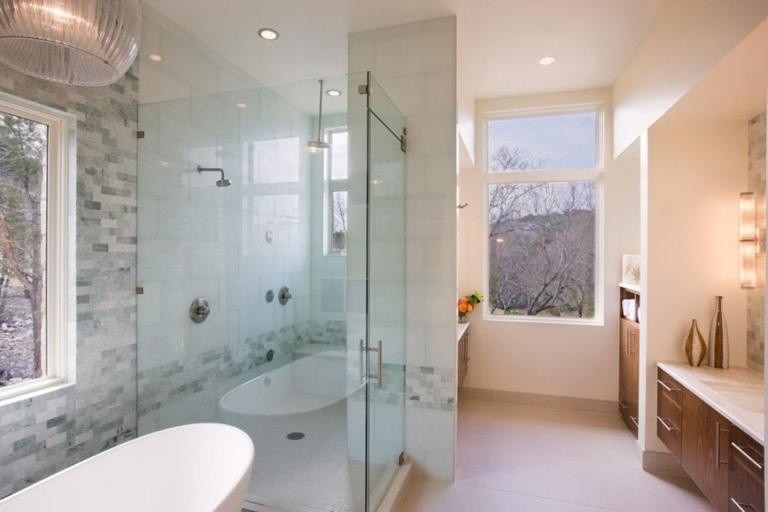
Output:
xmin=0 ymin=421 xmax=256 ymax=511
xmin=216 ymin=352 xmax=375 ymax=423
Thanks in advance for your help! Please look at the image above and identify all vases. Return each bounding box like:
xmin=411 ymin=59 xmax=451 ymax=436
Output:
xmin=458 ymin=312 xmax=469 ymax=323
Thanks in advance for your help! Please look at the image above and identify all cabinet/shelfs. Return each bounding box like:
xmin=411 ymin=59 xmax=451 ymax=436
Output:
xmin=458 ymin=321 xmax=471 ymax=392
xmin=656 ymin=361 xmax=764 ymax=512
xmin=619 ymin=282 xmax=640 ymax=440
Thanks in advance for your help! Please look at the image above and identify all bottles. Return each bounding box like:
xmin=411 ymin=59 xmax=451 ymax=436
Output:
xmin=684 ymin=295 xmax=731 ymax=370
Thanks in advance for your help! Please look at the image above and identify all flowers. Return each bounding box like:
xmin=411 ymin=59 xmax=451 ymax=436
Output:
xmin=458 ymin=291 xmax=484 ymax=313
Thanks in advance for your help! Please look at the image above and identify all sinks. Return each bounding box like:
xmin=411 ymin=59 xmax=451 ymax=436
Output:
xmin=700 ymin=379 xmax=766 ymax=414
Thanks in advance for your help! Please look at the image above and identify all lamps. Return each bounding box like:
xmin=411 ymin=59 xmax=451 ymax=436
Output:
xmin=1 ymin=1 xmax=142 ymax=87
xmin=741 ymin=192 xmax=765 ymax=290
xmin=307 ymin=80 xmax=330 ymax=154
